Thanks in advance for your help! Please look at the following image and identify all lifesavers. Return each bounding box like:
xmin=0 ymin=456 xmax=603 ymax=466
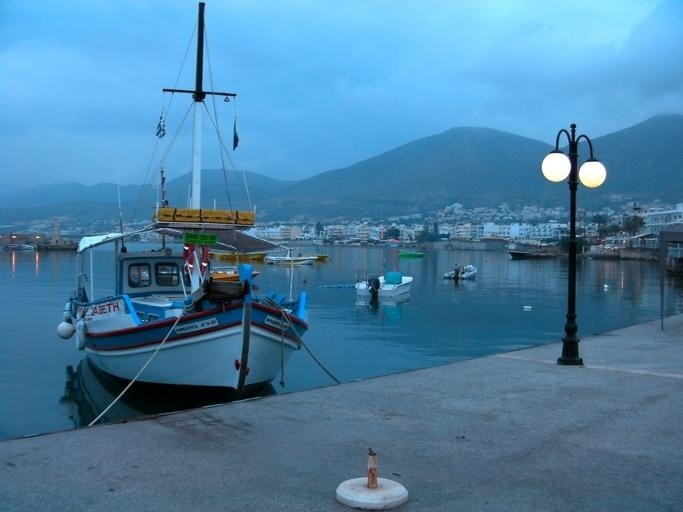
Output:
xmin=183 ymin=243 xmax=208 ymax=274
xmin=76 ymin=322 xmax=85 ymax=351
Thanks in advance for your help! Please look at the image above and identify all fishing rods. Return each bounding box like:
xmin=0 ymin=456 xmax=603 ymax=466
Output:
xmin=117 ymin=171 xmax=128 ymax=252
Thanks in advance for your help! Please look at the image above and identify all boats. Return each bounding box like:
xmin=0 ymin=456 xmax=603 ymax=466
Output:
xmin=263 ymin=254 xmax=328 ymax=265
xmin=443 ymin=265 xmax=477 ymax=280
xmin=354 ymin=271 xmax=413 ymax=297
xmin=55 ymin=0 xmax=309 ymax=391
xmin=397 ymin=250 xmax=425 ymax=258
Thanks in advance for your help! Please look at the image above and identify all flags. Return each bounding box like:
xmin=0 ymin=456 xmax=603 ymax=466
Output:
xmin=155 ymin=109 xmax=166 ymax=139
xmin=233 ymin=119 xmax=239 ymax=151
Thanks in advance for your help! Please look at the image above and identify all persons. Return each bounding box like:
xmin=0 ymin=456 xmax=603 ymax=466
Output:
xmin=454 ymin=263 xmax=459 ymax=274
xmin=373 ymin=277 xmax=380 ymax=290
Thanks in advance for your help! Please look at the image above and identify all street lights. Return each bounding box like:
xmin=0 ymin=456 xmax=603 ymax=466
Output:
xmin=540 ymin=122 xmax=606 ymax=367
